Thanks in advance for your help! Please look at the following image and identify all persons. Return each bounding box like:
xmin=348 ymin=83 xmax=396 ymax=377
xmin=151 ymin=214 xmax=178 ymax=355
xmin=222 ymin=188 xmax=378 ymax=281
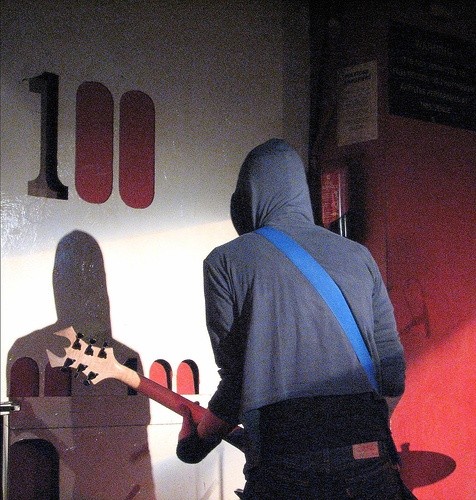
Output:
xmin=176 ymin=138 xmax=419 ymax=500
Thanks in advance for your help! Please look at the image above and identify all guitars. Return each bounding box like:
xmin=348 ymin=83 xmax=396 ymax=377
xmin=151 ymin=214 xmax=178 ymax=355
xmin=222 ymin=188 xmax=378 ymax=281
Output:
xmin=48 ymin=326 xmax=248 ymax=451
